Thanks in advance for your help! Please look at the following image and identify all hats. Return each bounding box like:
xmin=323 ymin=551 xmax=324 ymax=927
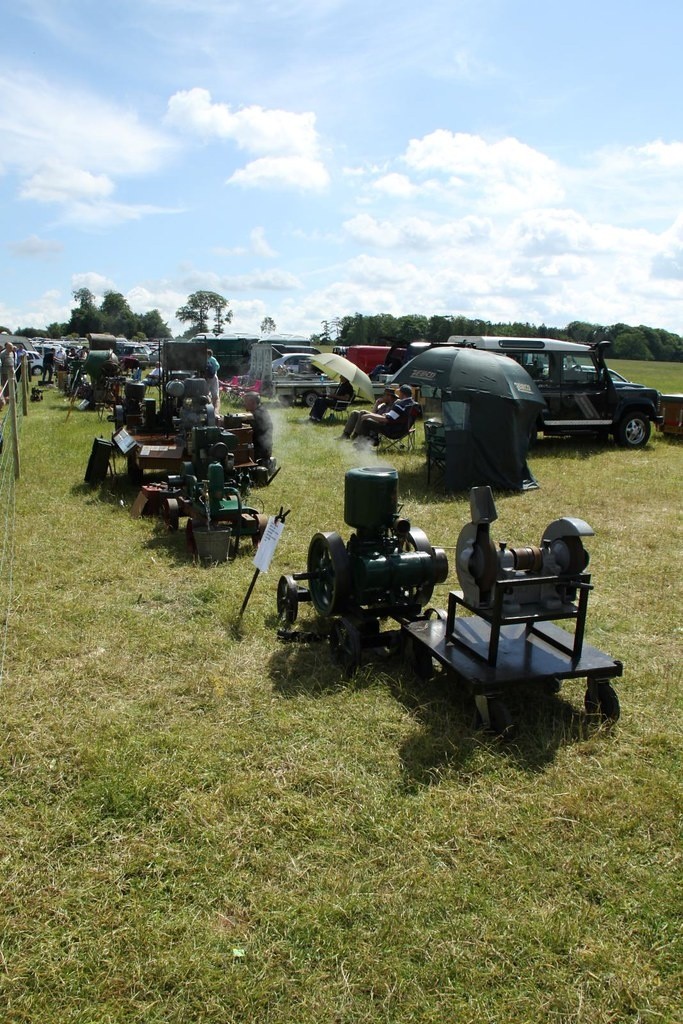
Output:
xmin=383 ymin=389 xmax=399 ymax=398
xmin=396 ymin=384 xmax=413 ymax=395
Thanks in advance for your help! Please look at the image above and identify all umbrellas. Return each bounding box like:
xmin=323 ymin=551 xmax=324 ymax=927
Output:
xmin=387 ymin=345 xmax=548 ymax=409
xmin=305 ymin=351 xmax=376 ymax=405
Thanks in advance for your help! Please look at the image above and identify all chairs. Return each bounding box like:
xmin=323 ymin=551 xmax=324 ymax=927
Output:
xmin=424 ymin=431 xmax=449 ymax=486
xmin=328 ymin=389 xmax=356 ymax=423
xmin=523 ymin=364 xmax=541 ymax=378
xmin=379 ymin=404 xmax=420 ymax=452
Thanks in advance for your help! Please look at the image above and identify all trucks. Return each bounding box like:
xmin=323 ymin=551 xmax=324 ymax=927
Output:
xmin=244 ymin=343 xmax=387 ymax=407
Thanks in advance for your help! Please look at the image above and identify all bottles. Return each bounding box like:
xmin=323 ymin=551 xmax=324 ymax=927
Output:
xmin=326 ymin=386 xmax=330 ymax=398
xmin=321 ymin=377 xmax=323 ymax=384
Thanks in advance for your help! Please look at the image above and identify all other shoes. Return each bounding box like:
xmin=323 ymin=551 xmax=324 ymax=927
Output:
xmin=309 ymin=417 xmax=320 ymax=424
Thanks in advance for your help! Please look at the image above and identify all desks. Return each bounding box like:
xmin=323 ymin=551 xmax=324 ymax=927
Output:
xmin=138 ymin=444 xmax=186 ymax=480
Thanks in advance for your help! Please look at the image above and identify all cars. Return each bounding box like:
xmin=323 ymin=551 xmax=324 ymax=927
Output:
xmin=20 ymin=350 xmax=45 ymax=377
xmin=151 ymin=342 xmax=160 ymax=362
xmin=28 ymin=338 xmax=88 ymax=349
xmin=117 ymin=342 xmax=150 ymax=371
xmin=272 ymin=353 xmax=317 ymax=370
xmin=332 ymin=344 xmax=406 ymax=374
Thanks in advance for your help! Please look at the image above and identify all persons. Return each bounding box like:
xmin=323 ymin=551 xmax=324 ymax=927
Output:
xmin=339 ymin=385 xmax=415 ymax=442
xmin=205 ymin=348 xmax=222 ymax=418
xmin=0 ymin=340 xmax=28 ymax=402
xmin=233 ymin=390 xmax=274 ymax=462
xmin=41 ymin=345 xmax=90 ymax=382
xmin=141 ymin=361 xmax=163 ymax=391
xmin=308 ymin=374 xmax=354 ymax=422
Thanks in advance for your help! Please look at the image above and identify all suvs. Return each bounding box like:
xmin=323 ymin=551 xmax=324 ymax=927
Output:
xmin=446 ymin=336 xmax=667 ymax=449
